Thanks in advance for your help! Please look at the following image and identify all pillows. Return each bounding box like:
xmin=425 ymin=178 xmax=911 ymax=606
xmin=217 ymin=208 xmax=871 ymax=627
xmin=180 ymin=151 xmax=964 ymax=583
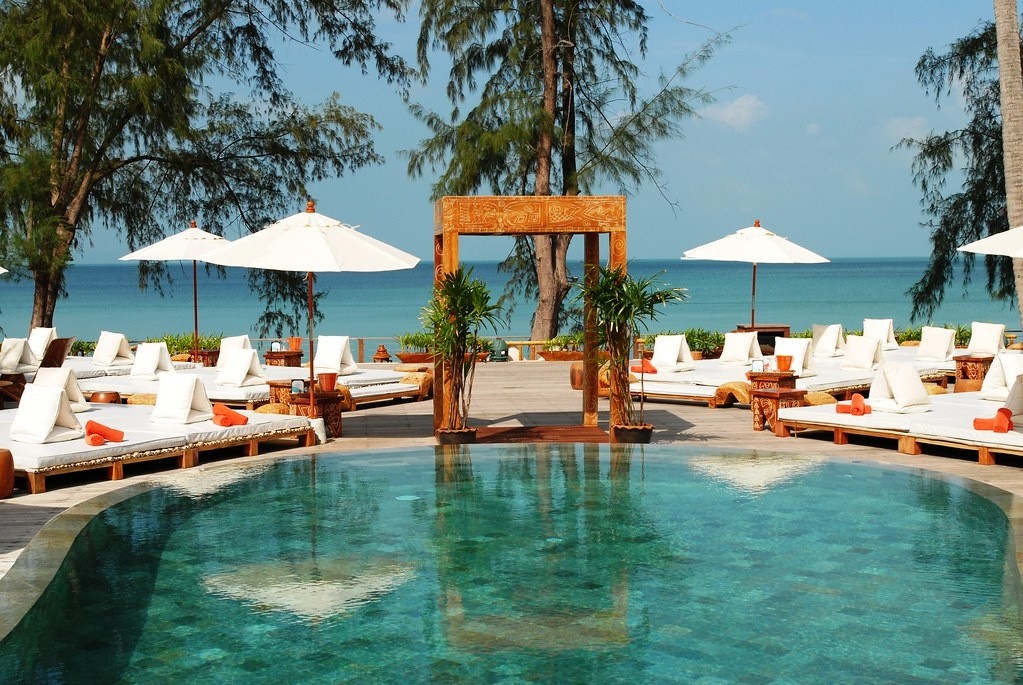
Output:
xmin=0 ymin=326 xmax=359 ymax=443
xmin=652 ymin=319 xmax=1023 ymax=417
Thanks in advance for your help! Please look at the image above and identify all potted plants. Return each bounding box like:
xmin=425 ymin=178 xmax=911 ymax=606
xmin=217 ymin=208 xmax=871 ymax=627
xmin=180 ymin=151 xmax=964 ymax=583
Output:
xmin=538 ymin=252 xmax=725 ymax=444
xmin=393 ymin=259 xmax=508 ymax=446
xmin=144 ymin=332 xmax=227 ymax=367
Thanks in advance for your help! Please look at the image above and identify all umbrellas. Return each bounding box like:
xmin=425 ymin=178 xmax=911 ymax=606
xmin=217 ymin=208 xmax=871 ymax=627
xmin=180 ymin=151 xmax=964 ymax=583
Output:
xmin=681 ymin=218 xmax=831 ymax=332
xmin=202 ymin=201 xmax=423 ymax=419
xmin=118 ymin=221 xmax=234 ymax=364
xmin=957 ymin=225 xmax=1023 ymax=261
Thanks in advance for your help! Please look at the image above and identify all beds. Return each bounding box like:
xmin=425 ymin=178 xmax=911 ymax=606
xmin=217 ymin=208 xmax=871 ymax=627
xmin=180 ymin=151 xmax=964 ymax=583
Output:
xmin=0 ymin=355 xmax=432 ymax=494
xmin=631 ymin=345 xmax=1023 ymax=466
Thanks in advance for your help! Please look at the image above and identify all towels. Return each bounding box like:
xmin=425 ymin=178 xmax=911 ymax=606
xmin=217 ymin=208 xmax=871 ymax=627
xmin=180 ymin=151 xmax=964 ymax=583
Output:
xmin=836 ymin=392 xmax=872 ymax=416
xmin=973 ymin=407 xmax=1014 ymax=433
xmin=83 ymin=419 xmax=125 ymax=446
xmin=631 ymin=357 xmax=658 ymax=374
xmin=212 ymin=402 xmax=249 ymax=426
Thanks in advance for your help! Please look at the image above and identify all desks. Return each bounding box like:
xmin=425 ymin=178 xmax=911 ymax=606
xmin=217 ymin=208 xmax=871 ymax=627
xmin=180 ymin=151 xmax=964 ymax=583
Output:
xmin=262 ymin=350 xmax=304 ymax=367
xmin=749 ymin=389 xmax=809 ymax=433
xmin=266 ymin=378 xmax=318 ymax=405
xmin=284 ymin=395 xmax=345 ymax=439
xmin=952 ymin=352 xmax=994 ymax=392
xmin=736 ymin=324 xmax=792 ymax=339
xmin=746 ymin=376 xmax=799 ymax=411
xmin=188 ymin=350 xmax=220 ymax=367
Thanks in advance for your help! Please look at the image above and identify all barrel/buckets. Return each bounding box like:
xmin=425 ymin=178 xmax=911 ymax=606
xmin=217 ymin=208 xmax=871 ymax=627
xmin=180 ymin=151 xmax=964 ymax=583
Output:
xmin=776 ymin=355 xmax=793 ymax=372
xmin=317 ymin=372 xmax=337 ymax=392
xmin=287 ymin=337 xmax=302 ymax=351
